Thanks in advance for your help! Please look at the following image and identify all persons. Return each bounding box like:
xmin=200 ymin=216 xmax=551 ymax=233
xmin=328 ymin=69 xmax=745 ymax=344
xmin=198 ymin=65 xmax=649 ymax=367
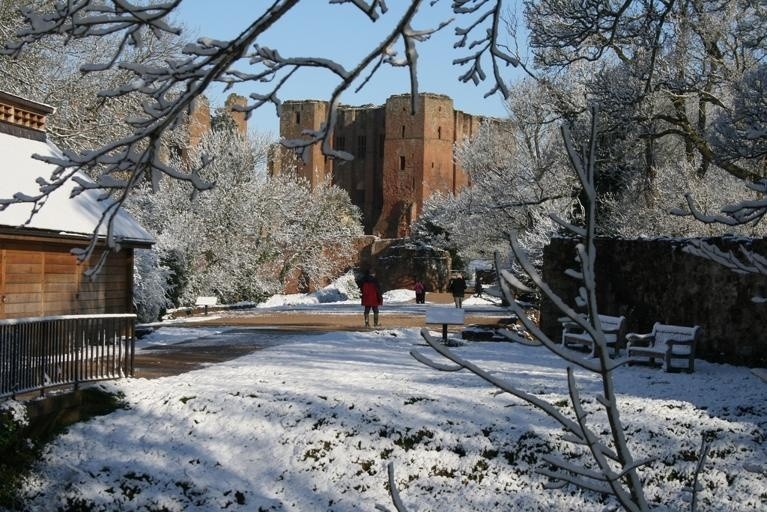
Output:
xmin=420 ymin=280 xmax=427 ymax=304
xmin=415 ymin=281 xmax=424 ymax=304
xmin=359 ymin=268 xmax=383 ymax=329
xmin=450 ymin=273 xmax=468 ymax=310
xmin=474 ymin=276 xmax=482 ymax=298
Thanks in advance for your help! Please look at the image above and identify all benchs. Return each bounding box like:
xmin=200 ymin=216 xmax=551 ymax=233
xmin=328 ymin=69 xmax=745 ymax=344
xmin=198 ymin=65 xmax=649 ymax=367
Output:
xmin=561 ymin=315 xmax=703 ymax=373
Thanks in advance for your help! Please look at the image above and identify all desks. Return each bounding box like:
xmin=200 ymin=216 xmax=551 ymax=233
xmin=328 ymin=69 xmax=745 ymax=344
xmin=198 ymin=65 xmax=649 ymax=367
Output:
xmin=425 ymin=306 xmax=464 ymax=347
